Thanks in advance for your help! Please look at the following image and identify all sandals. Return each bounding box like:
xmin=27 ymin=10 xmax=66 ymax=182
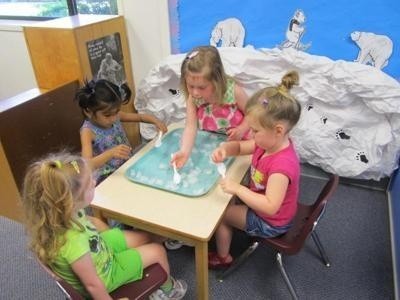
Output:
xmin=208 ymin=251 xmax=234 ymax=266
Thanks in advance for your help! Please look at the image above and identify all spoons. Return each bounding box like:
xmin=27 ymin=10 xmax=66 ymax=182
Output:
xmin=153 ymin=130 xmax=163 ymax=148
xmin=170 ymin=153 xmax=182 ymax=185
xmin=216 ymin=161 xmax=227 ymax=178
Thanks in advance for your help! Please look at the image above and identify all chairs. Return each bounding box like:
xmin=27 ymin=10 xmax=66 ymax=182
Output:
xmin=36 ymin=255 xmax=168 ymax=300
xmin=217 ymin=173 xmax=341 ymax=300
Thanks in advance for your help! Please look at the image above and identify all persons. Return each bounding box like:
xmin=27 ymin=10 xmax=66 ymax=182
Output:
xmin=20 ymin=151 xmax=188 ymax=299
xmin=170 ymin=45 xmax=253 ymax=175
xmin=206 ymin=71 xmax=302 ymax=270
xmin=99 ymin=53 xmax=121 ymax=83
xmin=76 ymin=80 xmax=184 ymax=250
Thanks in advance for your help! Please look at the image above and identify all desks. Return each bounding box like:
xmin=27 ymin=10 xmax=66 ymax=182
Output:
xmin=87 ymin=122 xmax=253 ymax=300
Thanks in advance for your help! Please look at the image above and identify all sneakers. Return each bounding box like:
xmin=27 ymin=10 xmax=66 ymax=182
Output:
xmin=165 ymin=239 xmax=194 ymax=249
xmin=149 ymin=279 xmax=188 ymax=300
